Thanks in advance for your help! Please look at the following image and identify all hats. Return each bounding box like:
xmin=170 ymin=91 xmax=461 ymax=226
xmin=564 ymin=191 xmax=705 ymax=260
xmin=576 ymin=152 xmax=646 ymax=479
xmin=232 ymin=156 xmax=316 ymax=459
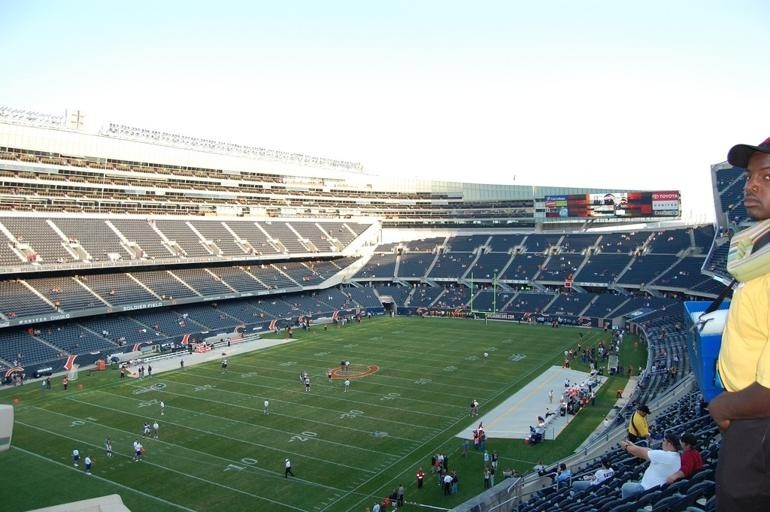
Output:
xmin=664 ymin=431 xmax=698 ymax=446
xmin=727 ymin=137 xmax=770 ymax=168
xmin=637 ymin=405 xmax=651 ymax=414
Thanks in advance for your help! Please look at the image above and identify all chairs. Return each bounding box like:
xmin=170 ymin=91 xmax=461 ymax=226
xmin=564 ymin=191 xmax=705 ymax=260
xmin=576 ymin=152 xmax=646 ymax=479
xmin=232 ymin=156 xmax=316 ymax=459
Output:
xmin=456 ymin=330 xmax=726 ymax=512
xmin=366 ymin=227 xmax=744 ymax=332
xmin=1 ymin=214 xmax=366 ymax=377
xmin=712 ymin=156 xmax=752 ymax=226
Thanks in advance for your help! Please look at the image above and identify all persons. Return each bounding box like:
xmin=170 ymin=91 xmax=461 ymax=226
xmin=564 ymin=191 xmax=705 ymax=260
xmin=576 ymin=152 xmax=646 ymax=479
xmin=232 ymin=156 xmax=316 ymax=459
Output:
xmin=0 ymin=204 xmax=744 ymax=512
xmin=707 ymin=140 xmax=770 ymax=511
